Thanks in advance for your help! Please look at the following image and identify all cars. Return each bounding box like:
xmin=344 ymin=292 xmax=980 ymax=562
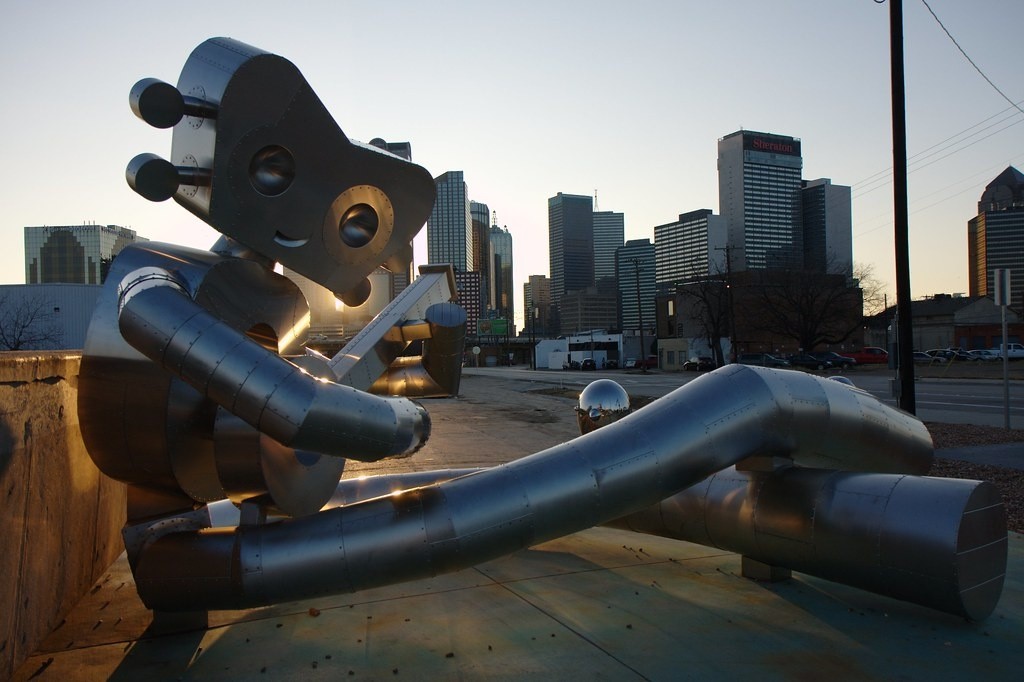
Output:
xmin=927 ymin=349 xmax=969 ymax=361
xmin=840 ymin=346 xmax=890 ymax=364
xmin=625 ymin=358 xmax=637 ymax=368
xmin=965 ymin=349 xmax=1003 ymax=364
xmin=823 ymin=352 xmax=857 ymax=370
xmin=913 ymin=351 xmax=947 ymax=364
xmin=605 ymin=359 xmax=618 ymax=369
xmin=786 ymin=354 xmax=831 ymax=370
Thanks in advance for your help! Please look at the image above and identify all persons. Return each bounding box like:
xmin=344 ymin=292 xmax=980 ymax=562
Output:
xmin=601 ymin=357 xmax=606 ymax=369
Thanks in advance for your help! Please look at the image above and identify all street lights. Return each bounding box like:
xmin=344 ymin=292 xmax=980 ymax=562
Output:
xmin=531 ymin=300 xmax=539 ymax=370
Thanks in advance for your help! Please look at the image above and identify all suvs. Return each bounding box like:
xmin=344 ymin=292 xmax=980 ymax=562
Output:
xmin=738 ymin=352 xmax=789 ymax=368
xmin=580 ymin=358 xmax=596 ymax=371
xmin=684 ymin=356 xmax=714 ymax=372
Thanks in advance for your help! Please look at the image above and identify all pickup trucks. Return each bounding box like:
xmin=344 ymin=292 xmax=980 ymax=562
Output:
xmin=987 ymin=343 xmax=1024 ymax=362
